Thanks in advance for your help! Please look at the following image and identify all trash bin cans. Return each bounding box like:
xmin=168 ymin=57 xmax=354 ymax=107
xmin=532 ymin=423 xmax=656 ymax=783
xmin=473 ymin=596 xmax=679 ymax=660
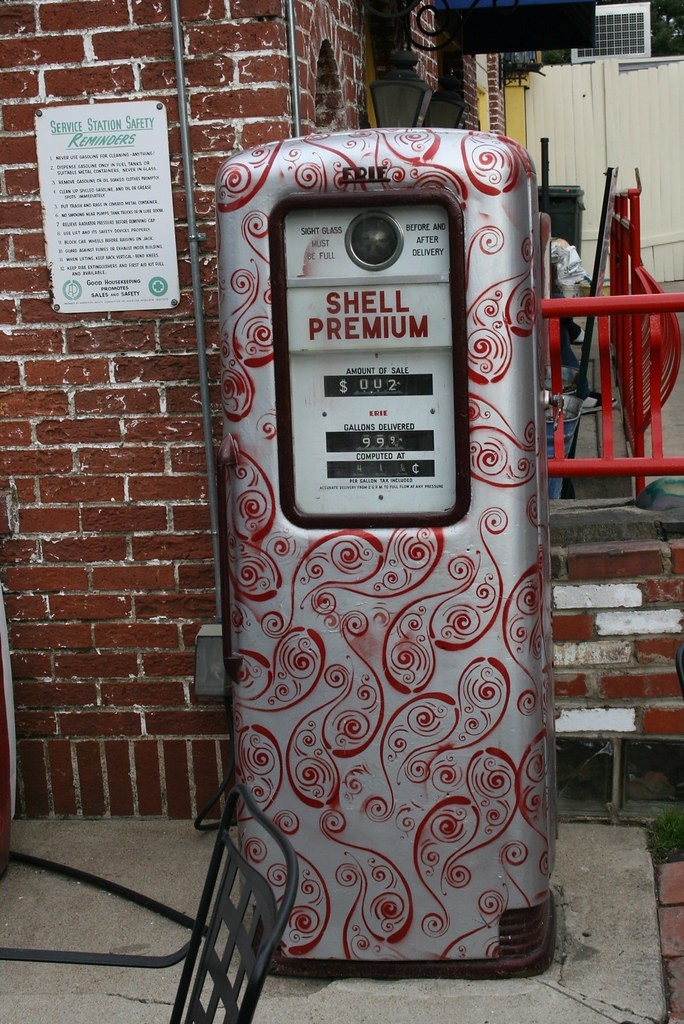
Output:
xmin=538 ymin=185 xmax=586 ymax=259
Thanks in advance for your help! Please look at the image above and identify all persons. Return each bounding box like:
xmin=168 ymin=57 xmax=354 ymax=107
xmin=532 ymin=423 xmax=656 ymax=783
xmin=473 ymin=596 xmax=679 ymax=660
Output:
xmin=550 ymin=216 xmax=616 ymax=497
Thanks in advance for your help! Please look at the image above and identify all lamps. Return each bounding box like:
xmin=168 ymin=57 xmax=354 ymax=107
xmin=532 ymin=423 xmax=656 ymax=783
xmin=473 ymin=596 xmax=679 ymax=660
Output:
xmin=370 ymin=40 xmax=434 ymax=128
xmin=498 ymin=52 xmax=531 ymax=89
xmin=422 ymin=69 xmax=471 ymax=128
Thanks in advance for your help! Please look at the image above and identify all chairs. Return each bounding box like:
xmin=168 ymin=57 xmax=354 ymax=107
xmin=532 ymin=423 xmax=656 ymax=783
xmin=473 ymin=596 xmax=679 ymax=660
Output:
xmin=170 ymin=784 xmax=299 ymax=1024
